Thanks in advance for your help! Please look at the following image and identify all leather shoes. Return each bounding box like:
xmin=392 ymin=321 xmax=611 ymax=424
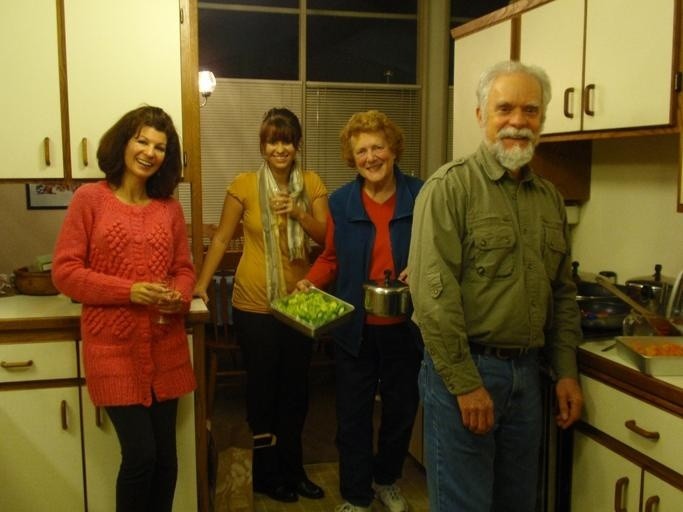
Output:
xmin=300 ymin=476 xmax=325 ymax=499
xmin=254 ymin=482 xmax=300 ymax=503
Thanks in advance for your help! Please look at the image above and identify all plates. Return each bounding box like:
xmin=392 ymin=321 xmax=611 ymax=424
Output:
xmin=613 ymin=336 xmax=683 ymax=377
xmin=269 ymin=286 xmax=356 ymax=337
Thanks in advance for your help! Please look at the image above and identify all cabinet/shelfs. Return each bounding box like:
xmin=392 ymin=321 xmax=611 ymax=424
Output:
xmin=557 ymin=332 xmax=683 ymax=512
xmin=0 ymin=277 xmax=208 ymax=512
xmin=452 ymin=3 xmax=588 ymax=204
xmin=513 ymin=0 xmax=683 ymax=145
xmin=1 ymin=0 xmax=185 ymax=183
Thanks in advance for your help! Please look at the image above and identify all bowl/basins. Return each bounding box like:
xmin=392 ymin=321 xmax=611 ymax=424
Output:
xmin=12 ymin=266 xmax=61 ymax=296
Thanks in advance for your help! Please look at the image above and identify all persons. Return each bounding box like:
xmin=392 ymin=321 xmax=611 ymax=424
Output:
xmin=295 ymin=110 xmax=429 ymax=512
xmin=52 ymin=106 xmax=195 ymax=512
xmin=408 ymin=63 xmax=586 ymax=512
xmin=194 ymin=107 xmax=329 ymax=512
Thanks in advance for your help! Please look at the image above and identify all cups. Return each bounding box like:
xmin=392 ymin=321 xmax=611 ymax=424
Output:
xmin=157 ymin=275 xmax=176 ymax=324
xmin=271 ymin=183 xmax=286 ymax=206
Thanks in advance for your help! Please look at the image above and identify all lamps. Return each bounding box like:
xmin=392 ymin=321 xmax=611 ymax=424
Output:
xmin=196 ymin=66 xmax=218 ymax=107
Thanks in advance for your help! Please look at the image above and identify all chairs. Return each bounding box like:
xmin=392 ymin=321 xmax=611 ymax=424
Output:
xmin=199 ymin=246 xmax=252 ymax=420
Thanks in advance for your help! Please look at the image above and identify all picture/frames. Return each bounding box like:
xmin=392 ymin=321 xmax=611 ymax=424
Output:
xmin=25 ymin=182 xmax=77 ymax=211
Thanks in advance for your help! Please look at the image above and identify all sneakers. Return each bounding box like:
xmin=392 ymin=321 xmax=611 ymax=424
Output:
xmin=374 ymin=481 xmax=409 ymax=512
xmin=335 ymin=500 xmax=375 ymax=511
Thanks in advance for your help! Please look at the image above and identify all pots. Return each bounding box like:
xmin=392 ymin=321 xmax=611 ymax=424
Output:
xmin=574 ymin=269 xmax=623 ymax=336
xmin=624 ymin=263 xmax=679 ymax=314
xmin=362 ymin=268 xmax=410 ymax=317
